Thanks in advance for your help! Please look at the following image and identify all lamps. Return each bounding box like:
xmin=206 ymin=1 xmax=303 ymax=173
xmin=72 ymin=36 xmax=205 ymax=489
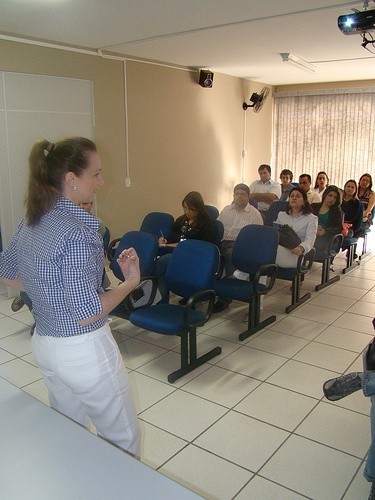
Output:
xmin=280 ymin=53 xmax=317 ymax=74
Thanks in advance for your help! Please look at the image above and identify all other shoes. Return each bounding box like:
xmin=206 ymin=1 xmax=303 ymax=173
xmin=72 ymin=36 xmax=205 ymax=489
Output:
xmin=329 ymin=264 xmax=336 ymax=272
xmin=178 ymin=298 xmax=197 ymax=304
xmin=213 ymin=298 xmax=232 ymax=313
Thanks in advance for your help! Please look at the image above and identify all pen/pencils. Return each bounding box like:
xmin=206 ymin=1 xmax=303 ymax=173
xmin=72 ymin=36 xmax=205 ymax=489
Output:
xmin=159 ymin=228 xmax=165 ymax=242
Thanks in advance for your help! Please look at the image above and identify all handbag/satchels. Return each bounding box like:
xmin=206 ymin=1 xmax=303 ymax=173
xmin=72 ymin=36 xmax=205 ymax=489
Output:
xmin=126 ymin=280 xmax=162 ymax=311
xmin=341 ymin=223 xmax=353 ymax=237
xmin=272 ymin=223 xmax=301 ymax=249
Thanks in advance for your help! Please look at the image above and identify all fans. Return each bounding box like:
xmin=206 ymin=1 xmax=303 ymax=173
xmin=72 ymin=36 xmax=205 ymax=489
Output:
xmin=241 ymin=86 xmax=271 ymax=114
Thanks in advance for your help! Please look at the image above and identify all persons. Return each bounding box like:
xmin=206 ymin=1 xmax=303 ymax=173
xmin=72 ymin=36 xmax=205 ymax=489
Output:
xmin=12 ymin=202 xmax=112 ymax=336
xmin=0 ymin=133 xmax=146 ymax=465
xmin=322 ymin=316 xmax=375 ymax=500
xmin=248 ymin=164 xmax=375 ymax=293
xmin=228 ymin=188 xmax=319 ymax=324
xmin=178 ymin=182 xmax=263 ymax=312
xmin=153 ymin=190 xmax=217 ymax=307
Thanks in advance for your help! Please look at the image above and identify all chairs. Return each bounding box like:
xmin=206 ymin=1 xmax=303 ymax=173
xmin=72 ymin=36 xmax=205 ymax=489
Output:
xmin=21 ymin=182 xmax=375 ymax=384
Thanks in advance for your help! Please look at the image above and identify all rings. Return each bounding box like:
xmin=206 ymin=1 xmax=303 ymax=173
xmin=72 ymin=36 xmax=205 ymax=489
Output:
xmin=129 ymin=253 xmax=132 ymax=256
xmin=127 ymin=254 xmax=130 ymax=258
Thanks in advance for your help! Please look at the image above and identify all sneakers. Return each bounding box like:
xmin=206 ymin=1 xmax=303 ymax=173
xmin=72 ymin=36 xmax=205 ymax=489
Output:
xmin=11 ymin=296 xmax=24 ymax=312
xmin=323 ymin=372 xmax=363 ymax=401
xmin=30 ymin=323 xmax=36 ymax=336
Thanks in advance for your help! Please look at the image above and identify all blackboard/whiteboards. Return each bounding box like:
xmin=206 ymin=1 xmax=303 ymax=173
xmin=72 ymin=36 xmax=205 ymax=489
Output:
xmin=0 ymin=71 xmax=98 ymax=257
